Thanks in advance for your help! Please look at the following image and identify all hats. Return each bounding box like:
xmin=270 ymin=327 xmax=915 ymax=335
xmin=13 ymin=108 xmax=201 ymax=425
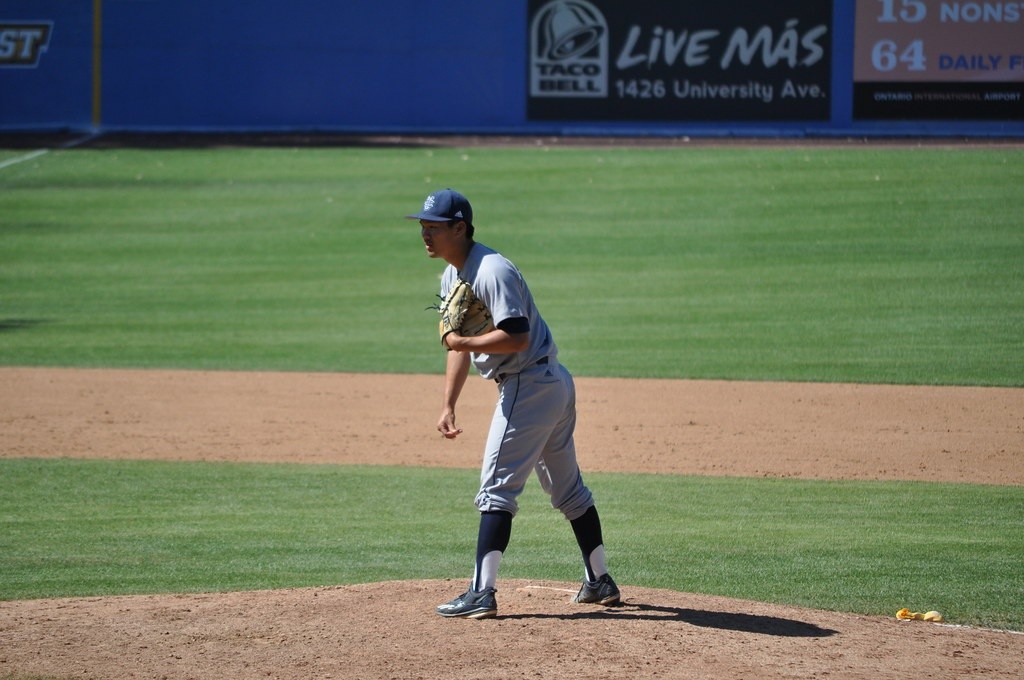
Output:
xmin=405 ymin=187 xmax=473 ymax=223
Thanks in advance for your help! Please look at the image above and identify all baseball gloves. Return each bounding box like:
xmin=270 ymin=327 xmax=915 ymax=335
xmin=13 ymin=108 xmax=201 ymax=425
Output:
xmin=424 ymin=276 xmax=494 ymax=353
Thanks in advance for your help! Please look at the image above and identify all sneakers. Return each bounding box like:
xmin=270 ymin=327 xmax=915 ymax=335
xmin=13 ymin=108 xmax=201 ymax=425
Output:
xmin=433 ymin=583 xmax=498 ymax=619
xmin=569 ymin=573 xmax=621 ymax=604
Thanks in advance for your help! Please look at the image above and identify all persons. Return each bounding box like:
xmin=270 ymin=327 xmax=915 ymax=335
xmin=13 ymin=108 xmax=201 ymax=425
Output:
xmin=403 ymin=187 xmax=622 ymax=620
